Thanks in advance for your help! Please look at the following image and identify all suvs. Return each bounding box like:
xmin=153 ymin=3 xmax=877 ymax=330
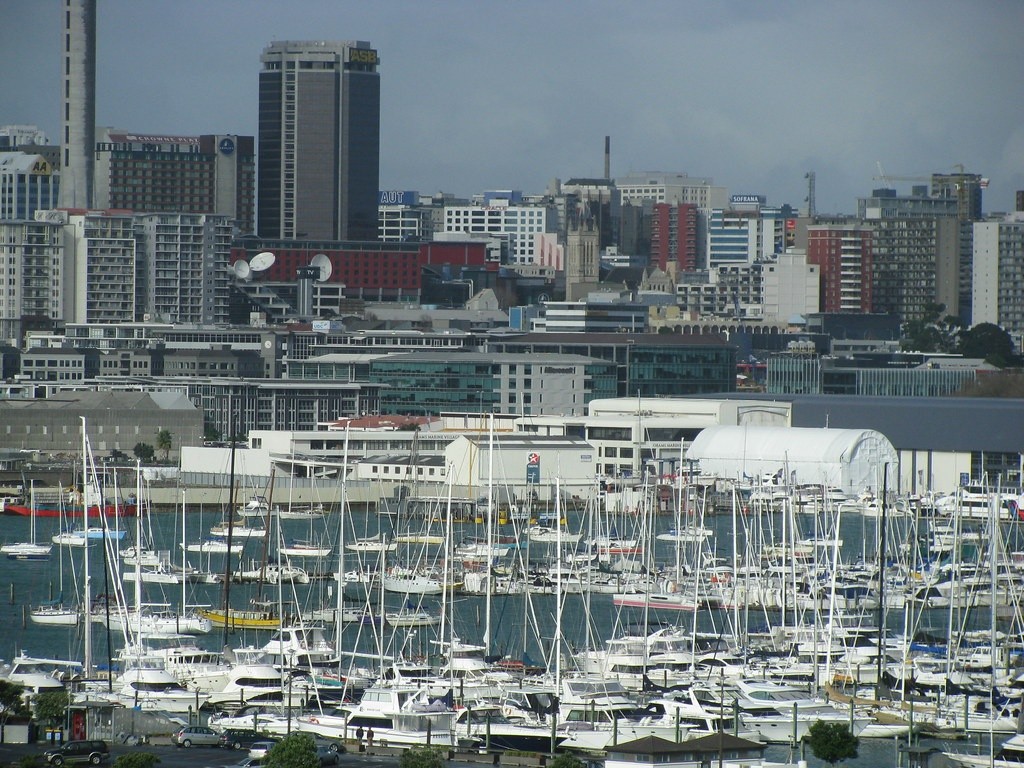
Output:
xmin=171 ymin=724 xmax=224 ymax=749
xmin=43 ymin=740 xmax=111 ymax=768
xmin=219 ymin=728 xmax=279 ymax=751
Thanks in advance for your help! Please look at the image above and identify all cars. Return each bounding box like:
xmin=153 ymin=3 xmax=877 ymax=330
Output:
xmin=313 ymin=746 xmax=340 ymax=768
xmin=248 ymin=741 xmax=280 ymax=765
xmin=283 ymin=730 xmax=341 ymax=753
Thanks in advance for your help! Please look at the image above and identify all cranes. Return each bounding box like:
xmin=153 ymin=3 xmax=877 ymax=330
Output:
xmin=870 ymin=162 xmax=990 ymax=224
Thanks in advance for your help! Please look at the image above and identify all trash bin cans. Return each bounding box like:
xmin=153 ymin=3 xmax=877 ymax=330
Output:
xmin=44 ymin=729 xmax=52 ymax=745
xmin=54 ymin=730 xmax=62 ymax=745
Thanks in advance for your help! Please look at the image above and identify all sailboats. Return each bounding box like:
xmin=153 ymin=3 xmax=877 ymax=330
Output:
xmin=0 ymin=389 xmax=1023 ymax=768
